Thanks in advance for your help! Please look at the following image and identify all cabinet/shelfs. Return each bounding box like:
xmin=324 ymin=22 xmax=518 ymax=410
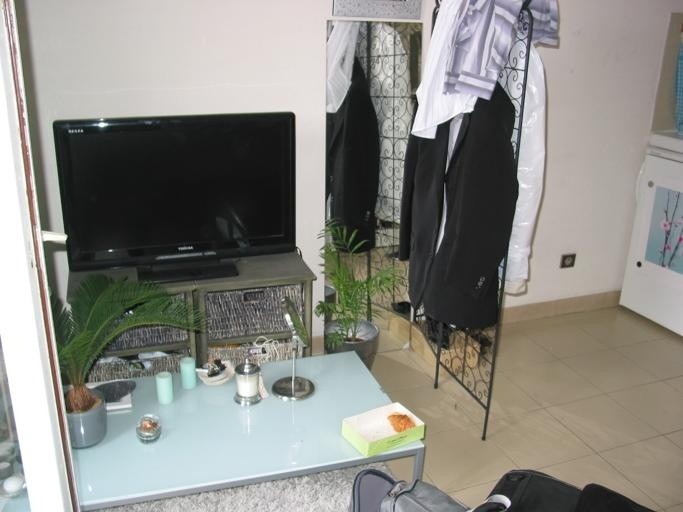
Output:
xmin=619 ymin=132 xmax=683 ymax=338
xmin=50 ymin=252 xmax=317 ymax=383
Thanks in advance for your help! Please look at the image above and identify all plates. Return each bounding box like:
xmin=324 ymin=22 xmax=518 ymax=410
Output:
xmin=197 ymin=360 xmax=235 ymax=387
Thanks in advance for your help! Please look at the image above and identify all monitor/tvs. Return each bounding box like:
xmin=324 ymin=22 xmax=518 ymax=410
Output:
xmin=52 ymin=112 xmax=297 ymax=286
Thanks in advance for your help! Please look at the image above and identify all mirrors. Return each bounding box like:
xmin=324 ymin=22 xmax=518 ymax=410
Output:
xmin=271 ymin=296 xmax=314 ymax=401
xmin=326 ymin=21 xmax=422 ymax=353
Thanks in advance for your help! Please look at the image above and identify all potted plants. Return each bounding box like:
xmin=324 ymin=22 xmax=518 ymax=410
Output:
xmin=314 ymin=217 xmax=406 ymax=371
xmin=46 ymin=277 xmax=209 ymax=448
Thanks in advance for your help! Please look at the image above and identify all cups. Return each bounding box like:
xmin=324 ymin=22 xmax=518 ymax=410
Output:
xmin=179 ymin=357 xmax=197 ymax=390
xmin=155 ymin=371 xmax=174 ymax=405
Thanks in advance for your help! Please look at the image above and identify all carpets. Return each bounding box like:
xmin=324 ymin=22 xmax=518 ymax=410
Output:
xmin=80 ymin=461 xmax=397 ymax=512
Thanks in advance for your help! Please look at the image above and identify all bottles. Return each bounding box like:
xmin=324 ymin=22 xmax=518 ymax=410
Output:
xmin=234 ymin=357 xmax=262 ymax=408
